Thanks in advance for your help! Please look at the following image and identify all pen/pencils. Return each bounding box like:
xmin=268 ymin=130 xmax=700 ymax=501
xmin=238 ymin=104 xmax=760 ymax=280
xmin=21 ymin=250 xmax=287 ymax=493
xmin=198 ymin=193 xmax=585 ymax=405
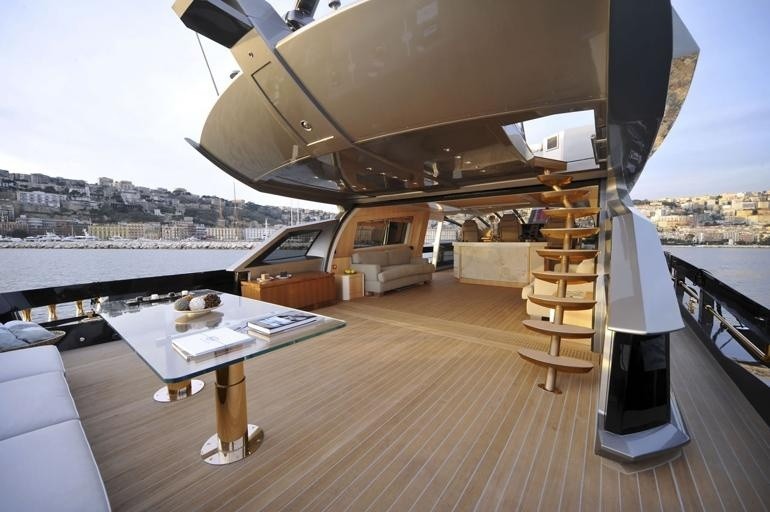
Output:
xmin=171 ymin=343 xmax=190 ymax=362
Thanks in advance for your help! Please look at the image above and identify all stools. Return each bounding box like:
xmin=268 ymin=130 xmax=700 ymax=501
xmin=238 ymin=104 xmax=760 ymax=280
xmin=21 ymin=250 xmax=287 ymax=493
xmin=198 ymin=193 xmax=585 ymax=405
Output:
xmin=0 ymin=330 xmax=65 ymax=353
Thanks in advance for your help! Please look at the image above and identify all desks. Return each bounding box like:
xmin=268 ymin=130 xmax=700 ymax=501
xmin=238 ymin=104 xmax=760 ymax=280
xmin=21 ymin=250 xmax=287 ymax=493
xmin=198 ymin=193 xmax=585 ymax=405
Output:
xmin=90 ymin=289 xmax=346 ymax=465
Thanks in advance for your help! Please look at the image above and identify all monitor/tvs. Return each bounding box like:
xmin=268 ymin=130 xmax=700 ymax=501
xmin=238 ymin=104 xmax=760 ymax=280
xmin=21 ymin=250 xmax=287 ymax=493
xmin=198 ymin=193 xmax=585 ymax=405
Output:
xmin=521 ymin=223 xmax=544 ymax=239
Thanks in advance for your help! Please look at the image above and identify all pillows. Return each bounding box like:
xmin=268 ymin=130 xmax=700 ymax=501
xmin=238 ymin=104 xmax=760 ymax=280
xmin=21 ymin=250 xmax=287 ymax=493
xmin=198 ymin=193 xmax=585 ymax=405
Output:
xmin=4 ymin=320 xmax=55 ymax=343
xmin=0 ymin=323 xmax=28 ymax=351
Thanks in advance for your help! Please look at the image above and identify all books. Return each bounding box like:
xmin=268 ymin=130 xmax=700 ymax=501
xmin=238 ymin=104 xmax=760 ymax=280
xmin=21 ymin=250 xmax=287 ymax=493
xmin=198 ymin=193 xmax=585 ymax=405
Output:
xmin=247 ymin=317 xmax=325 ymax=343
xmin=172 ymin=334 xmax=256 ymax=360
xmin=246 ymin=310 xmax=317 ymax=334
xmin=172 ymin=327 xmax=249 ymax=356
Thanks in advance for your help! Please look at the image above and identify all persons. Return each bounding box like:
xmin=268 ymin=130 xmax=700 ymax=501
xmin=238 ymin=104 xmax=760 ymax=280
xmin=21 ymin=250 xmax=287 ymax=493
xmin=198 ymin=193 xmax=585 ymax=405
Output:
xmin=485 ymin=214 xmax=498 ymax=235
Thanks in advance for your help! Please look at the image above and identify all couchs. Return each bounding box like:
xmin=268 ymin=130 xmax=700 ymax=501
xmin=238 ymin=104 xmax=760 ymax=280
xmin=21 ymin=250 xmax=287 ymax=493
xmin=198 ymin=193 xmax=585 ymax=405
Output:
xmin=349 ymin=244 xmax=435 ymax=297
xmin=521 ymin=259 xmax=595 ymax=316
xmin=0 ymin=344 xmax=112 ymax=512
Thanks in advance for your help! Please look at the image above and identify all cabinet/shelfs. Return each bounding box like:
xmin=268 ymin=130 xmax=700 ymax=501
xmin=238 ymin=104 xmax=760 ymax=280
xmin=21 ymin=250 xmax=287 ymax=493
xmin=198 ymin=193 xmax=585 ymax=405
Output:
xmin=334 ymin=272 xmax=365 ymax=301
xmin=240 ymin=270 xmax=337 ymax=310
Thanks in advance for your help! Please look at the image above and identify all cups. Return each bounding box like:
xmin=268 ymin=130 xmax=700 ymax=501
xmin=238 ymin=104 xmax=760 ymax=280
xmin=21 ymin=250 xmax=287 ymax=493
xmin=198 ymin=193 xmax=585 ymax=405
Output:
xmin=261 ymin=273 xmax=269 ymax=278
xmin=281 ymin=271 xmax=287 ymax=277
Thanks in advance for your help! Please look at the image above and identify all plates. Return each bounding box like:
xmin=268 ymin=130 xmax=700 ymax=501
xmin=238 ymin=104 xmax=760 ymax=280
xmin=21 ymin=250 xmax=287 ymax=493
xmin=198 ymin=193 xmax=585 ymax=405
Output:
xmin=171 ymin=312 xmax=224 ymax=325
xmin=259 ymin=278 xmax=275 ymax=282
xmin=170 ymin=302 xmax=225 ymax=312
xmin=276 ymin=275 xmax=292 ymax=279
